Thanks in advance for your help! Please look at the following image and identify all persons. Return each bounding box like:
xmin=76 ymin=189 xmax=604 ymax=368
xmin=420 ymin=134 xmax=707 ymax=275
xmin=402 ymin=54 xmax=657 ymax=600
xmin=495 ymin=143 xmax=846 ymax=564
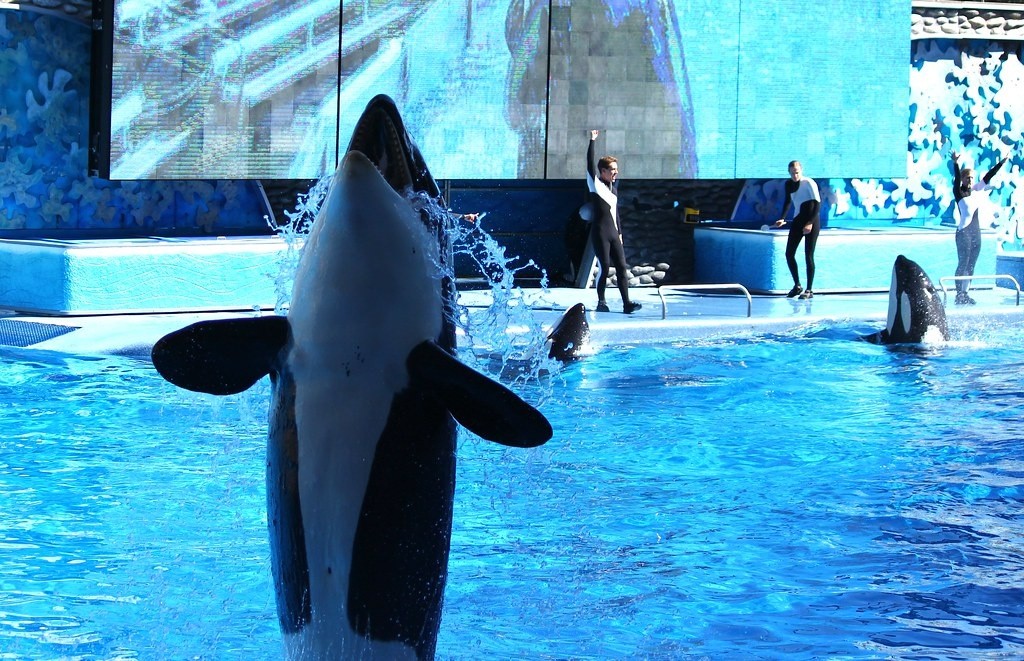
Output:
xmin=948 ymin=148 xmax=1009 ymax=304
xmin=586 ymin=130 xmax=642 ymax=314
xmin=776 ymin=161 xmax=821 ymax=299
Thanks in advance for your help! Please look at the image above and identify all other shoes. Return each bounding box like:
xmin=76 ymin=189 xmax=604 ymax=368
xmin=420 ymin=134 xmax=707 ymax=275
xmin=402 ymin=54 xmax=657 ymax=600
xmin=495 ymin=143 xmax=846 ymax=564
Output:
xmin=597 ymin=303 xmax=610 ymax=312
xmin=798 ymin=290 xmax=813 ymax=300
xmin=954 ymin=293 xmax=976 ymax=304
xmin=623 ymin=301 xmax=642 ymax=313
xmin=787 ymin=285 xmax=803 ymax=297
